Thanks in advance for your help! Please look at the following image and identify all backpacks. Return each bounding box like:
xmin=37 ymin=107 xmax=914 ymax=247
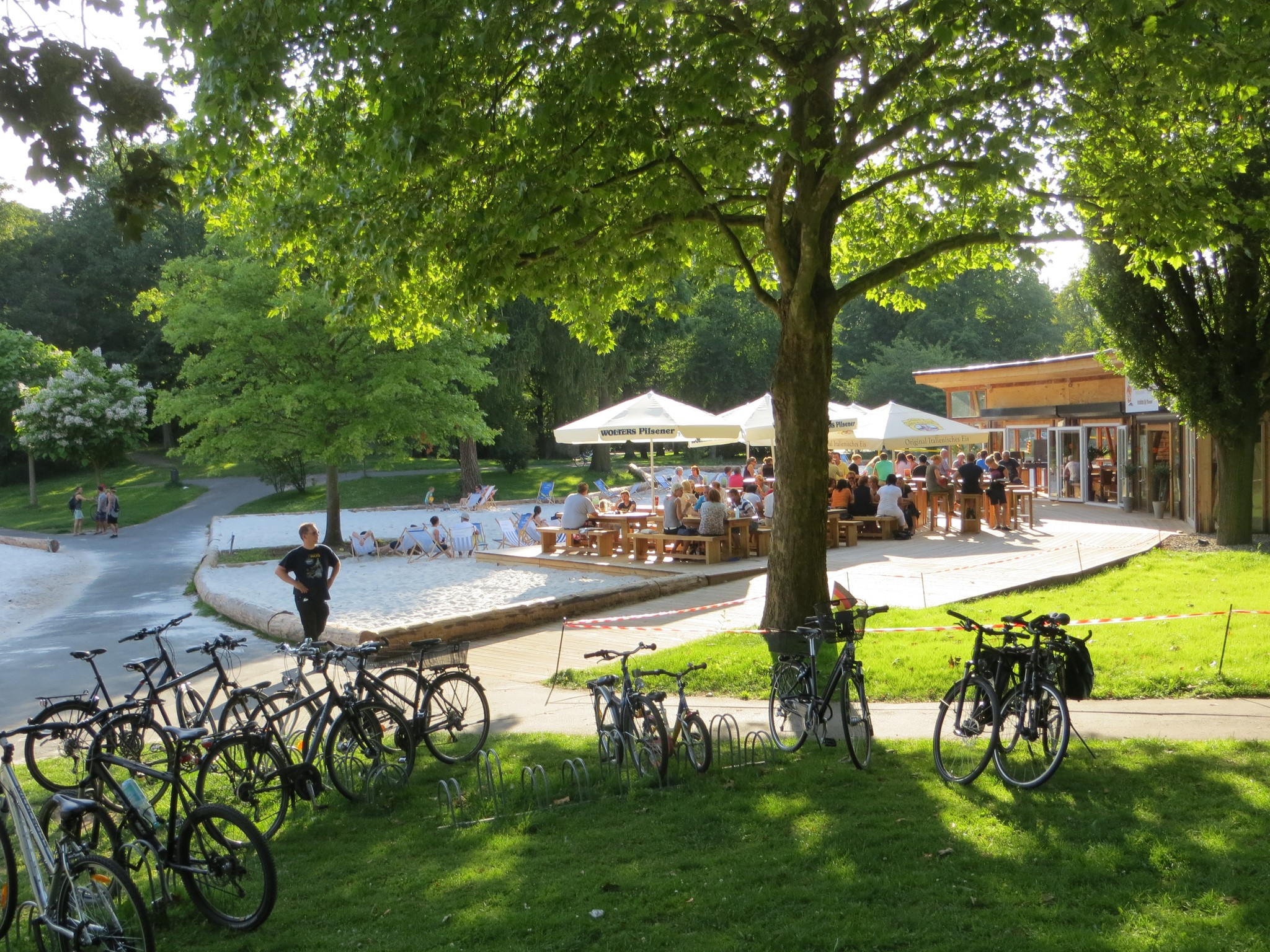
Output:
xmin=68 ymin=493 xmax=80 ymax=510
xmin=110 ymin=495 xmax=120 ymax=512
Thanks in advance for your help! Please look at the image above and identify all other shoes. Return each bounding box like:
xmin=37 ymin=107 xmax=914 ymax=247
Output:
xmin=689 ymin=548 xmax=694 ymax=555
xmin=949 ymin=502 xmax=960 ymax=517
xmin=79 ymin=533 xmax=86 ymax=535
xmin=701 ymin=548 xmax=706 ymax=555
xmin=965 ymin=509 xmax=975 ymax=519
xmin=993 ymin=526 xmax=1013 ymax=530
xmin=1017 ymin=500 xmax=1019 ymax=505
xmin=110 ymin=534 xmax=118 ymax=539
xmin=94 ymin=531 xmax=102 ymax=535
xmin=308 ymin=654 xmax=329 ymax=672
xmin=101 ymin=531 xmax=108 ymax=535
xmin=909 ymin=529 xmax=915 ymax=536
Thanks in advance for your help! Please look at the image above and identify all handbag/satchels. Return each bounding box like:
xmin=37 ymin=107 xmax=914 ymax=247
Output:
xmin=892 ymin=529 xmax=911 ymax=540
xmin=985 ymin=487 xmax=993 ymax=496
xmin=938 ymin=478 xmax=948 ymax=486
xmin=1066 ymin=642 xmax=1095 ymax=700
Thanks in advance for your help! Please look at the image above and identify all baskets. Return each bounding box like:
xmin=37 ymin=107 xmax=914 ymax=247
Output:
xmin=765 ymin=626 xmax=825 ymax=657
xmin=364 ymin=641 xmax=469 ymax=669
xmin=814 ymin=598 xmax=869 ymax=643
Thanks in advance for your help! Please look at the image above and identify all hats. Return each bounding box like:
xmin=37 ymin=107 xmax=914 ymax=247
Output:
xmin=109 ymin=486 xmax=117 ymax=492
xmin=98 ymin=486 xmax=103 ymax=491
xmin=459 ymin=512 xmax=470 ymax=520
xmin=100 ymin=484 xmax=106 ymax=488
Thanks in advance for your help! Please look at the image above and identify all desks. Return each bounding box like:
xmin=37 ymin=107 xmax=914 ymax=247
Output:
xmin=829 ymin=501 xmax=910 ymax=531
xmin=635 ymin=505 xmax=665 ymax=515
xmin=744 ymin=477 xmax=775 ymax=488
xmin=763 ymin=509 xmax=847 ymax=548
xmin=647 ymin=516 xmax=752 ymax=561
xmin=557 ymin=512 xmax=656 ymax=554
xmin=1060 ymin=472 xmax=1116 ymax=498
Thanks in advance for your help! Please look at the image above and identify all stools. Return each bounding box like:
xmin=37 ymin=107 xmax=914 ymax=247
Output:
xmin=984 ymin=488 xmax=1010 ymax=528
xmin=931 ymin=493 xmax=951 ymax=531
xmin=911 ymin=488 xmax=926 ymax=527
xmin=1012 ymin=489 xmax=1032 ymax=529
xmin=959 ymin=494 xmax=982 ymax=533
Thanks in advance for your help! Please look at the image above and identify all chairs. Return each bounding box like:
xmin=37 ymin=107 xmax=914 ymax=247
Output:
xmin=534 ymin=475 xmax=671 ymax=504
xmin=348 ymin=512 xmax=567 ymax=563
xmin=456 ymin=485 xmax=498 ymax=512
xmin=1071 ymin=469 xmax=1118 ymax=498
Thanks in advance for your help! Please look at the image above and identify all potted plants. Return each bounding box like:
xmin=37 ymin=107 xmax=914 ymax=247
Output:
xmin=1122 ymin=464 xmax=1136 ymax=512
xmin=1087 ymin=445 xmax=1115 ymax=502
xmin=1153 ymin=461 xmax=1170 ymax=519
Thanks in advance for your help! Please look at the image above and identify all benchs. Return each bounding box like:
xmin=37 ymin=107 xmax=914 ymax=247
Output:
xmin=535 ymin=517 xmax=897 ymax=564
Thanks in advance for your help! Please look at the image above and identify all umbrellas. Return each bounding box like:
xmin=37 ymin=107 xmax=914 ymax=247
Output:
xmin=553 ymin=390 xmax=741 ymax=450
xmin=688 ymin=393 xmax=858 ymax=450
xmin=823 ymin=397 xmax=990 ymax=454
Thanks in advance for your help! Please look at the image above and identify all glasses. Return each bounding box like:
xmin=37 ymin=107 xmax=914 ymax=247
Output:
xmin=691 ymin=470 xmax=695 ymax=471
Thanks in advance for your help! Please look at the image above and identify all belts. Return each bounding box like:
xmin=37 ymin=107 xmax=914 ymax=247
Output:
xmin=765 ymin=516 xmax=772 ymax=518
xmin=664 ymin=524 xmax=682 ymax=530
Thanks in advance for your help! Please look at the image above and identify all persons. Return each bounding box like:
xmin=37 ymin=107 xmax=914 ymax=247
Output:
xmin=530 ymin=505 xmax=548 ymax=532
xmin=827 ymin=449 xmax=1025 ymax=539
xmin=451 ymin=512 xmax=480 ymax=559
xmin=612 ymin=490 xmax=636 ymax=548
xmin=560 ymin=482 xmax=596 ymax=554
xmin=1061 ymin=454 xmax=1081 ymax=498
xmin=92 ymin=485 xmax=110 ymax=535
xmin=369 ymin=524 xmax=418 ymax=555
xmin=662 ymin=456 xmax=774 ymax=562
xmin=423 ymin=516 xmax=451 ymax=554
xmin=458 ymin=485 xmax=489 ymax=511
xmin=350 ymin=529 xmax=375 ymax=554
xmin=440 ymin=500 xmax=451 ymax=512
xmin=108 ymin=487 xmax=119 ymax=538
xmin=68 ymin=486 xmax=92 ymax=535
xmin=274 ymin=522 xmax=342 ymax=671
xmin=424 ymin=486 xmax=436 ymax=512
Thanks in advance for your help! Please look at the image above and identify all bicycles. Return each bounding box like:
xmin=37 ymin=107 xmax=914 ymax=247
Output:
xmin=584 ymin=643 xmax=669 ymax=784
xmin=0 ymin=632 xmax=492 ymax=952
xmin=638 ymin=661 xmax=714 ymax=773
xmin=89 ymin=497 xmax=122 ymax=521
xmin=932 ymin=607 xmax=1097 ymax=789
xmin=24 ymin=612 xmax=215 ymax=793
xmin=574 ymin=450 xmax=594 ymax=468
xmin=768 ymin=597 xmax=890 ymax=770
